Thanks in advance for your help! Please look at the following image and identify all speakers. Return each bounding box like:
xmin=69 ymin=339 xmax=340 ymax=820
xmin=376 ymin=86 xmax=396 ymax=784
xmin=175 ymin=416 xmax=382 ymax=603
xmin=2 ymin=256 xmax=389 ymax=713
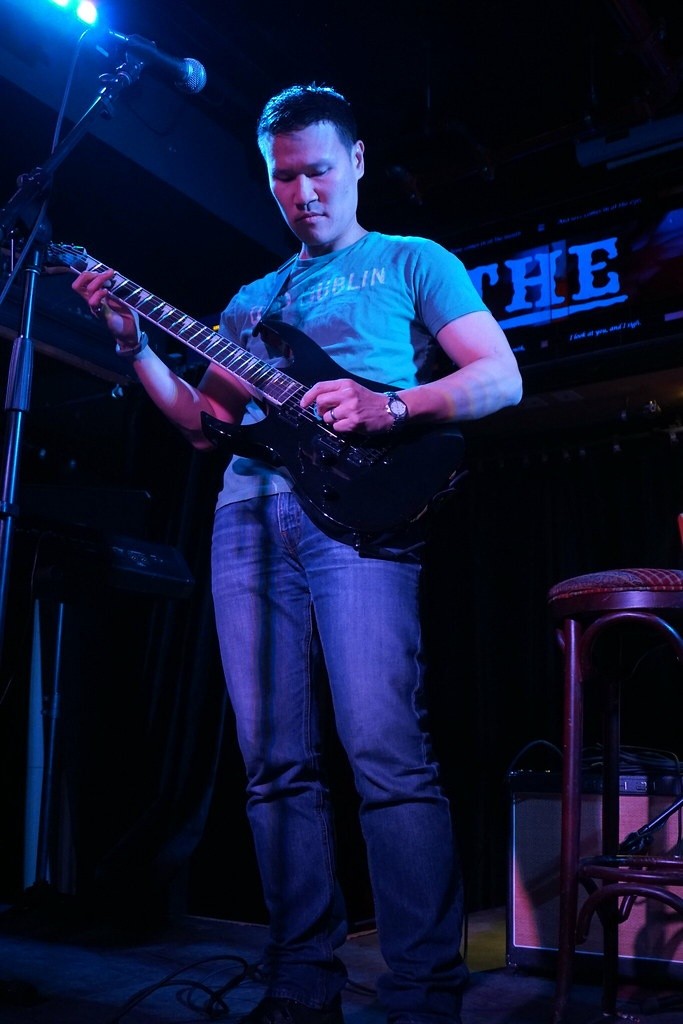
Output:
xmin=504 ymin=786 xmax=683 ymax=985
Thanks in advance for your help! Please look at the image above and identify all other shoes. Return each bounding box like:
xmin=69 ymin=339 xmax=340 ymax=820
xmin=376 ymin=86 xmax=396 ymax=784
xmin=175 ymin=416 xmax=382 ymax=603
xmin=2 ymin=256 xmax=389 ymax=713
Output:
xmin=237 ymin=992 xmax=344 ymax=1024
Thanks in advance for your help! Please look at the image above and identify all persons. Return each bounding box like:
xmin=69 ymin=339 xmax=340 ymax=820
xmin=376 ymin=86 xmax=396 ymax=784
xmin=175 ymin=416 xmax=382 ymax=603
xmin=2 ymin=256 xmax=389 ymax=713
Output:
xmin=69 ymin=82 xmax=524 ymax=1024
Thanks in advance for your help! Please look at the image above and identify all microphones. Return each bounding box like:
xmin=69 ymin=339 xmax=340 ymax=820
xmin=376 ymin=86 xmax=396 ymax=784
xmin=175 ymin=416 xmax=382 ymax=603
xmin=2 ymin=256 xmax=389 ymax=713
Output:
xmin=104 ymin=30 xmax=207 ymax=94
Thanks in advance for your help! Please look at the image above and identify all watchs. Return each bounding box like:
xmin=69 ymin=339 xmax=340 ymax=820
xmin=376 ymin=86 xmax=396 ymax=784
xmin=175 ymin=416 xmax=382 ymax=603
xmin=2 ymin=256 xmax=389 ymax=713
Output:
xmin=384 ymin=390 xmax=408 ymax=432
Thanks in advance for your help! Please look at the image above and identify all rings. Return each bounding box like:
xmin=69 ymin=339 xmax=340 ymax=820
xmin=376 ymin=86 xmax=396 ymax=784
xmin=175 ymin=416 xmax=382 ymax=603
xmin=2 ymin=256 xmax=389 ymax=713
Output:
xmin=91 ymin=304 xmax=104 ymax=313
xmin=329 ymin=409 xmax=337 ymax=421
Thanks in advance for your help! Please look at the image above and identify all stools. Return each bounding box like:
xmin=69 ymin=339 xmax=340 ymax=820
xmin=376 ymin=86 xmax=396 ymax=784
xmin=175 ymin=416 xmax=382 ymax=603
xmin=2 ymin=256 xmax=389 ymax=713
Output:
xmin=549 ymin=568 xmax=683 ymax=1024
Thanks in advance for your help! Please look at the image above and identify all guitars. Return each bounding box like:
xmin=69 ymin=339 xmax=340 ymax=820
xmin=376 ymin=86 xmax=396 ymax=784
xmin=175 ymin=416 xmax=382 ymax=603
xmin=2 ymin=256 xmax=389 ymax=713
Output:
xmin=47 ymin=238 xmax=478 ymax=555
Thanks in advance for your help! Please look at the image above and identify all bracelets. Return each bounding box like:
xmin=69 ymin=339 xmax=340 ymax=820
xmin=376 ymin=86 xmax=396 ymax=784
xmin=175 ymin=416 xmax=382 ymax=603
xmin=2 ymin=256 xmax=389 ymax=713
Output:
xmin=114 ymin=332 xmax=149 ymax=357
